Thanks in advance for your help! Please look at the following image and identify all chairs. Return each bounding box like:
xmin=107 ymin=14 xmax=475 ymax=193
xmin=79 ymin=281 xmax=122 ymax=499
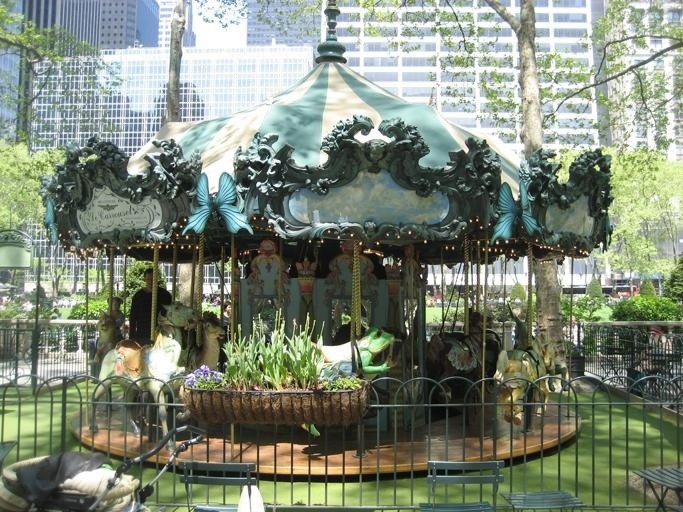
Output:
xmin=418 ymin=459 xmax=506 ymax=512
xmin=177 ymin=459 xmax=258 ymax=512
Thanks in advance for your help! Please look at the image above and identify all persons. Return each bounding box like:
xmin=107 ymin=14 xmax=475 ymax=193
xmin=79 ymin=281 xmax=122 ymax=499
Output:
xmin=221 ymin=301 xmax=231 ymax=340
xmin=634 ymin=325 xmax=674 ymax=373
xmin=463 ymin=312 xmax=482 ymax=334
xmin=562 ymin=315 xmax=585 ymax=345
xmin=129 ymin=268 xmax=172 ymax=339
xmin=511 ymin=307 xmax=535 ymax=347
xmin=100 ymin=297 xmax=125 ymax=340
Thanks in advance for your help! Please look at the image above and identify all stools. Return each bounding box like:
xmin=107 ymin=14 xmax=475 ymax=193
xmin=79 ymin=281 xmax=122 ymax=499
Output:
xmin=630 ymin=464 xmax=682 ymax=512
xmin=497 ymin=489 xmax=585 ymax=512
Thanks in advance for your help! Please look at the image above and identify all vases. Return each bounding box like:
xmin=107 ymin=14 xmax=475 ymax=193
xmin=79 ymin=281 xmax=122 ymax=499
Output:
xmin=181 ymin=383 xmax=371 ymax=427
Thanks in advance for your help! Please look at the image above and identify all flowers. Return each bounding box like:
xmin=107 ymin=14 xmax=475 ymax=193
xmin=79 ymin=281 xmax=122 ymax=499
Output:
xmin=180 ymin=308 xmax=370 ymax=391
